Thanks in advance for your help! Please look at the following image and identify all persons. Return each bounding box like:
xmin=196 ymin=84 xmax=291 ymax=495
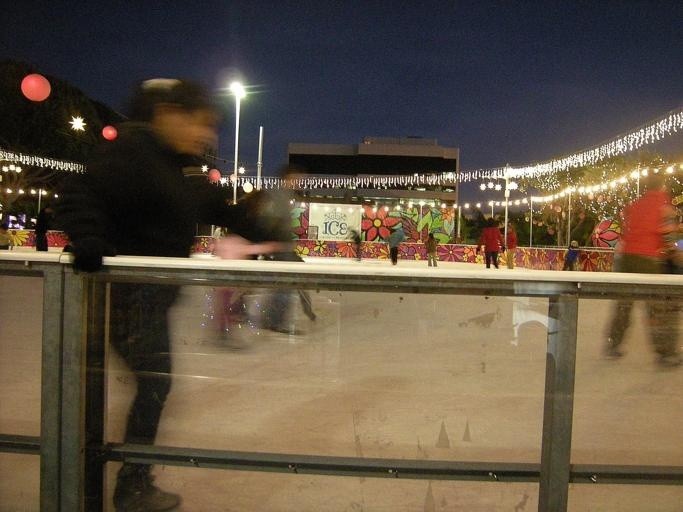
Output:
xmin=197 ymin=229 xmax=299 ymax=354
xmin=32 ymin=204 xmax=57 ymax=250
xmin=258 ymin=173 xmax=317 ymax=338
xmin=424 ymin=232 xmax=437 ymax=267
xmin=506 ymin=224 xmax=517 ymax=269
xmin=598 ymin=175 xmax=682 ymax=370
xmin=349 ymin=229 xmax=362 ymax=261
xmin=209 ymin=224 xmax=227 ymax=258
xmin=474 ymin=217 xmax=505 ymax=269
xmin=561 ymin=240 xmax=579 ymax=270
xmin=64 ymin=76 xmax=302 ymax=511
xmin=225 ymin=189 xmax=274 ymax=260
xmin=384 ymin=229 xmax=403 ymax=265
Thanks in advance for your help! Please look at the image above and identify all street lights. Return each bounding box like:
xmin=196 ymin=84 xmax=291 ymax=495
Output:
xmin=226 ymin=78 xmax=245 ymax=204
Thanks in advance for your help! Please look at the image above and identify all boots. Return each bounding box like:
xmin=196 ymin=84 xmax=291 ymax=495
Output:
xmin=113 ymin=471 xmax=180 ymax=512
